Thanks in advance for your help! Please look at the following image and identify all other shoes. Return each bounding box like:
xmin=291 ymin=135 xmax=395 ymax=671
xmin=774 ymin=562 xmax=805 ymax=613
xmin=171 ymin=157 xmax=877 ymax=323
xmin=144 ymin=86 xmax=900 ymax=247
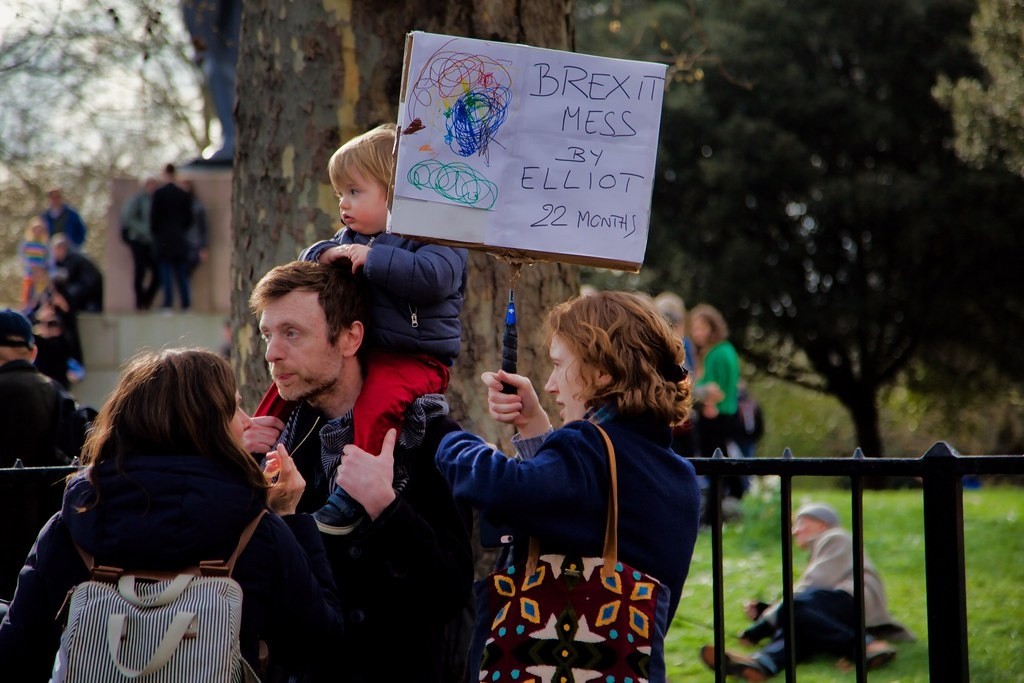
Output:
xmin=699 ymin=644 xmax=770 ymax=683
xmin=311 ymin=486 xmax=367 ymax=535
xmin=838 ymin=639 xmax=897 ymax=672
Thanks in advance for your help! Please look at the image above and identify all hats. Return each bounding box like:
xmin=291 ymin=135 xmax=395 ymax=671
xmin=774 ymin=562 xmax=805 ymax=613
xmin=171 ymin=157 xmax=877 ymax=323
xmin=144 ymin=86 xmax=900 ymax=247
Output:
xmin=51 ymin=234 xmax=66 ymax=247
xmin=0 ymin=308 xmax=35 ymax=348
xmin=795 ymin=504 xmax=840 ymax=529
xmin=45 ymin=185 xmax=61 ymax=194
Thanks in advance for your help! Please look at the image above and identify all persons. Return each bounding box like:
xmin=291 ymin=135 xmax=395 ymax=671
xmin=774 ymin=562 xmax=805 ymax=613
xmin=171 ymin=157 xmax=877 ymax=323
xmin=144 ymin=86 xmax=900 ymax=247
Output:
xmin=238 ymin=121 xmax=471 ymax=683
xmin=20 ymin=163 xmax=210 ymax=393
xmin=700 ymin=502 xmax=906 ymax=683
xmin=0 ymin=309 xmax=98 ymax=601
xmin=656 ymin=288 xmax=765 ymax=520
xmin=0 ymin=351 xmax=346 ymax=683
xmin=425 ymin=291 xmax=701 ymax=683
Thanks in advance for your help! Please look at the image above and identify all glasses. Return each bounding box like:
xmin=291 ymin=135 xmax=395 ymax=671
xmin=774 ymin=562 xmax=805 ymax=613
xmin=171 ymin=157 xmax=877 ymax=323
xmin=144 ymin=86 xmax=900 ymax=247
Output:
xmin=31 ymin=318 xmax=59 ymax=329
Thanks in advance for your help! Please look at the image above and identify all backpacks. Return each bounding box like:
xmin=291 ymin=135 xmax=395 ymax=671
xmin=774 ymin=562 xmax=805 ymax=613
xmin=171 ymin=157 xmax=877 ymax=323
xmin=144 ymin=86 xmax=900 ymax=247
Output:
xmin=48 ymin=510 xmax=270 ymax=683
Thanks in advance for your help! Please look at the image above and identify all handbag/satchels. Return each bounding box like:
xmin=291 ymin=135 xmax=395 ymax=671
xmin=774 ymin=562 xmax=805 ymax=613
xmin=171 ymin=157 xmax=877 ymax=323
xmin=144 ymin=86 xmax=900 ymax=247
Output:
xmin=476 ymin=417 xmax=662 ymax=683
xmin=119 ymin=195 xmax=142 ymax=244
